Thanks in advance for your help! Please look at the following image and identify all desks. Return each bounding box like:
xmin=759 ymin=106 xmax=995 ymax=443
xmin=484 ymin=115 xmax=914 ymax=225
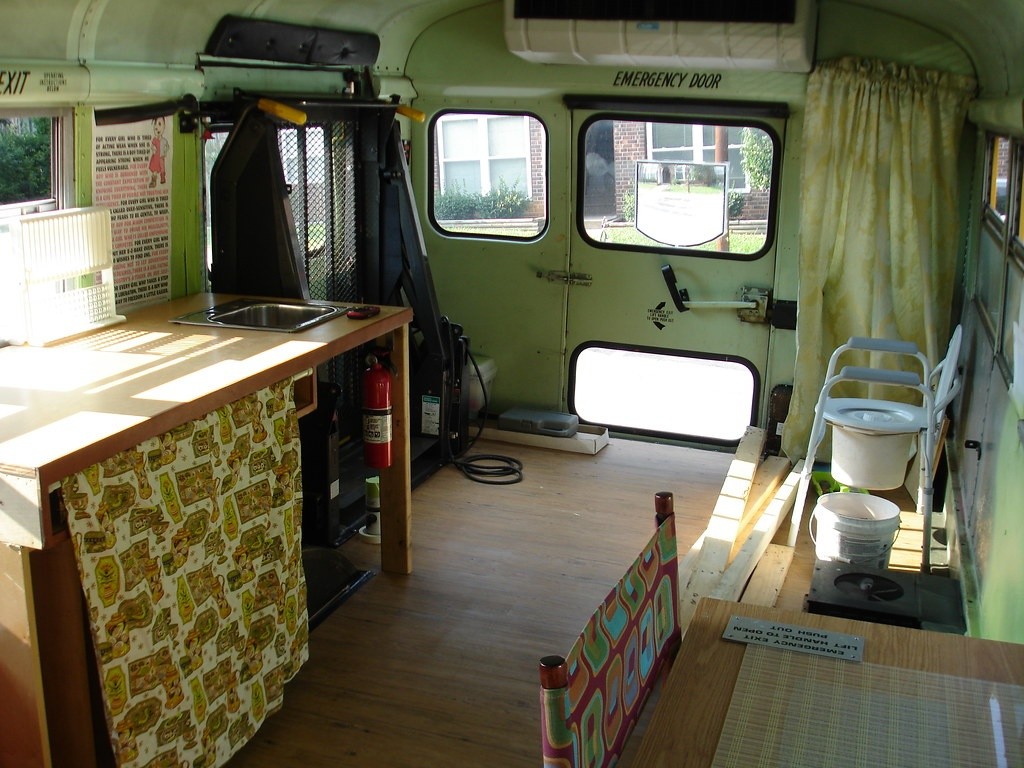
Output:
xmin=608 ymin=598 xmax=1024 ymax=768
xmin=0 ymin=292 xmax=413 ymax=768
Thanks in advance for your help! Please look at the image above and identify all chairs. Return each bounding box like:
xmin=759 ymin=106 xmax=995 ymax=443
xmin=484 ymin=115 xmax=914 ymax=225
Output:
xmin=540 ymin=492 xmax=683 ymax=768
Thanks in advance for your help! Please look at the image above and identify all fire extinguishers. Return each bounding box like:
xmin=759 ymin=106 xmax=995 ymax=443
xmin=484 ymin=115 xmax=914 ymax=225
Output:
xmin=361 ymin=352 xmax=398 ymax=468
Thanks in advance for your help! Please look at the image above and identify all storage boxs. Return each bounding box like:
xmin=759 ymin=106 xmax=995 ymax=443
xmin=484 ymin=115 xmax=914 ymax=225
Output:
xmin=464 ymin=355 xmax=498 ymax=417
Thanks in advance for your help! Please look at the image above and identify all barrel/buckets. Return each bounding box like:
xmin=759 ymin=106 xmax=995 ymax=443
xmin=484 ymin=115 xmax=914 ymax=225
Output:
xmin=809 ymin=492 xmax=902 ymax=568
xmin=825 ymin=420 xmax=917 ymax=491
